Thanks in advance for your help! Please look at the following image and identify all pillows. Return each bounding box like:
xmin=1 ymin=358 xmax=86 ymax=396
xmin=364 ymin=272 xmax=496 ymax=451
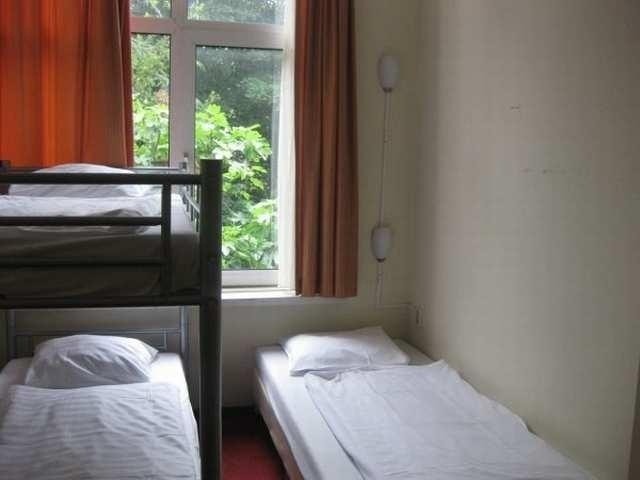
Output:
xmin=24 ymin=334 xmax=159 ymax=389
xmin=278 ymin=325 xmax=411 ymax=377
xmin=7 ymin=163 xmax=153 ymax=197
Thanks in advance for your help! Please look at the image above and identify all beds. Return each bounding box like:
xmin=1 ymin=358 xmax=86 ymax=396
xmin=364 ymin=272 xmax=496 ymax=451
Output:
xmin=252 ymin=338 xmax=600 ymax=480
xmin=0 ymin=160 xmax=222 ymax=480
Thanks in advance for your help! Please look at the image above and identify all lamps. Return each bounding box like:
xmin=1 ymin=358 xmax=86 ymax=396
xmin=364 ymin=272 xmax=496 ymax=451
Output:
xmin=370 ymin=55 xmax=422 ymax=328
